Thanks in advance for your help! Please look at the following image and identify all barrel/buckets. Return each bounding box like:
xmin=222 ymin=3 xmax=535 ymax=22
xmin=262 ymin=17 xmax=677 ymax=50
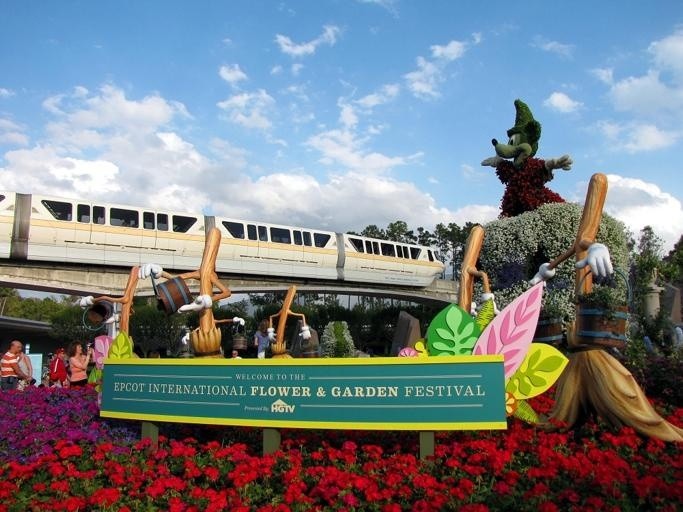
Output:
xmin=81 ymin=302 xmax=114 ymax=331
xmin=150 ymin=272 xmax=192 ymax=317
xmin=233 ymin=323 xmax=248 ymax=350
xmin=535 ymin=315 xmax=565 ymax=351
xmin=574 ymin=267 xmax=630 ymax=347
xmin=300 ymin=338 xmax=319 ymax=360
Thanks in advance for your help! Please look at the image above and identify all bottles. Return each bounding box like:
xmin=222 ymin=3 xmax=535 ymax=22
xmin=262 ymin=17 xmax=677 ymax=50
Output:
xmin=25 ymin=344 xmax=30 ymax=354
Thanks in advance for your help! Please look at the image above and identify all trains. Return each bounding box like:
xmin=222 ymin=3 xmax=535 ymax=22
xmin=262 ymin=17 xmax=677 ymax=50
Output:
xmin=0 ymin=192 xmax=446 ymax=286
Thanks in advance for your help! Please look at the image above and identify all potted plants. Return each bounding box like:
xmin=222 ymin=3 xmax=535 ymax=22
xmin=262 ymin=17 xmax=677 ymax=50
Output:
xmin=232 ymin=333 xmax=248 ymax=350
xmin=531 ymin=287 xmax=575 ymax=348
xmin=302 ymin=343 xmax=318 ymax=359
xmin=576 ymin=287 xmax=628 ymax=351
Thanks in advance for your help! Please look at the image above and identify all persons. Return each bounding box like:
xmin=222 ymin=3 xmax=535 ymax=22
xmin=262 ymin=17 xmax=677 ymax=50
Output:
xmin=0 ymin=331 xmax=192 ymax=392
xmin=628 ymin=315 xmax=683 ymax=356
xmin=229 ymin=349 xmax=242 ymax=359
xmin=252 ymin=318 xmax=272 ymax=359
